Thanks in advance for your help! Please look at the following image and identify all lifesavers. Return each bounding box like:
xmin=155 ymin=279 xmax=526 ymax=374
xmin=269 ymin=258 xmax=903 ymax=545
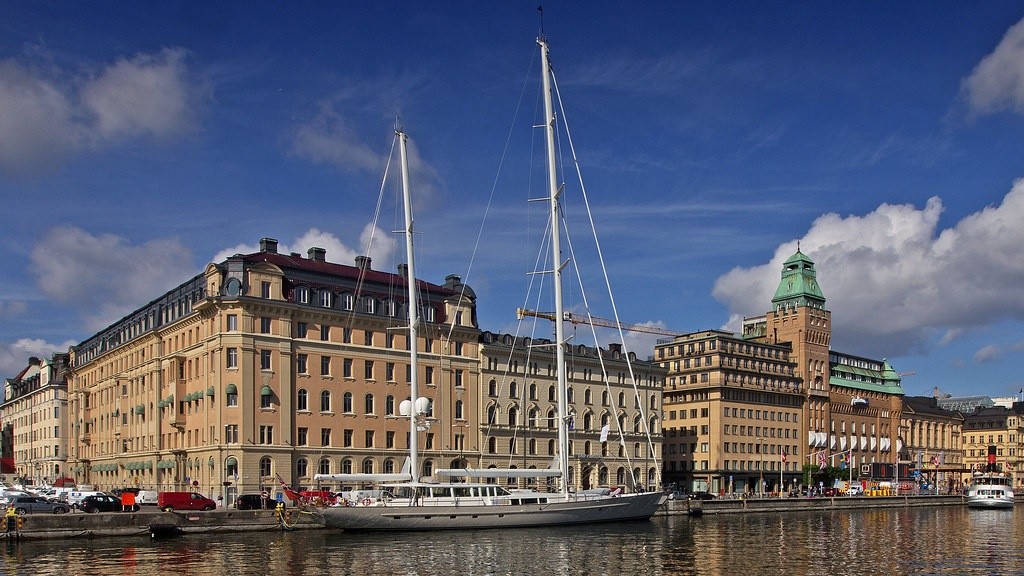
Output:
xmin=361 ymin=499 xmax=372 ymax=507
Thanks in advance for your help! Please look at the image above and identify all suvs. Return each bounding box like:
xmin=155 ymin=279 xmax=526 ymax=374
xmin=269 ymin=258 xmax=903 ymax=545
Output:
xmin=79 ymin=495 xmax=140 ymax=513
xmin=233 ymin=494 xmax=286 ymax=510
xmin=8 ymin=496 xmax=70 ymax=516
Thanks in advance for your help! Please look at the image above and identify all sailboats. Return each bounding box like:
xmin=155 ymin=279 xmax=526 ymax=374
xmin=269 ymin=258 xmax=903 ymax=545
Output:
xmin=297 ymin=31 xmax=670 ymax=527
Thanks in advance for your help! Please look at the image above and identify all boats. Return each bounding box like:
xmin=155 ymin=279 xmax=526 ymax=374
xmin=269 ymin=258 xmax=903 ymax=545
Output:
xmin=967 ymin=444 xmax=1016 ymax=508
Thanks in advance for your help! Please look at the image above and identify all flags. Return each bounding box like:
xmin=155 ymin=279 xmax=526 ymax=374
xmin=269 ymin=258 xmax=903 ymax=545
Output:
xmin=599 ymin=424 xmax=610 ymax=442
xmin=817 ymin=450 xmax=828 ymax=469
xmin=781 ymin=448 xmax=787 ymax=463
xmin=838 ymin=450 xmax=850 ymax=471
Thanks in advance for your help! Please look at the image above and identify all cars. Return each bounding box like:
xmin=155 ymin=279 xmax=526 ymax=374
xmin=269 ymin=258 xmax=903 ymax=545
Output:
xmin=687 ymin=490 xmax=717 ymax=500
xmin=824 ymin=486 xmax=844 ymax=497
xmin=665 ymin=490 xmax=689 ymax=500
xmin=0 ymin=485 xmax=121 ymax=510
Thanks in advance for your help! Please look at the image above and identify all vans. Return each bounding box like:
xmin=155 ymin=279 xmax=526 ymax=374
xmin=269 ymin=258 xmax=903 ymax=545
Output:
xmin=297 ymin=491 xmax=338 ymax=505
xmin=157 ymin=491 xmax=217 ymax=512
xmin=133 ymin=491 xmax=158 ymax=505
xmin=846 ymin=485 xmax=864 ymax=495
